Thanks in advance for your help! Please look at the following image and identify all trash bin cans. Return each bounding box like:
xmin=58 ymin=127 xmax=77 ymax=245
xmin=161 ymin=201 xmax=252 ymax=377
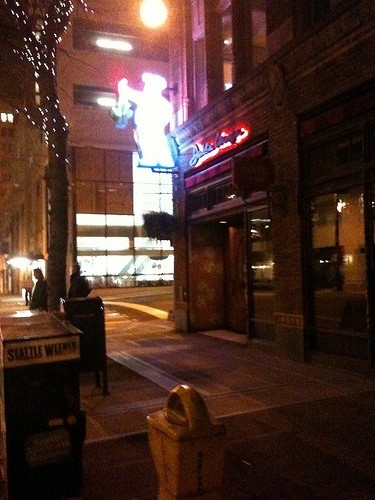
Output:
xmin=62 ymin=296 xmax=109 ymax=396
xmin=0 ymin=308 xmax=87 ymax=500
xmin=146 ymin=384 xmax=227 ymax=500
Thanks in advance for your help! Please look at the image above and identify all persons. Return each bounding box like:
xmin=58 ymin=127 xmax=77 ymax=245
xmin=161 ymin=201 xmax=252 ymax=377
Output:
xmin=28 ymin=268 xmax=49 ymax=312
xmin=67 ymin=262 xmax=92 ymax=300
